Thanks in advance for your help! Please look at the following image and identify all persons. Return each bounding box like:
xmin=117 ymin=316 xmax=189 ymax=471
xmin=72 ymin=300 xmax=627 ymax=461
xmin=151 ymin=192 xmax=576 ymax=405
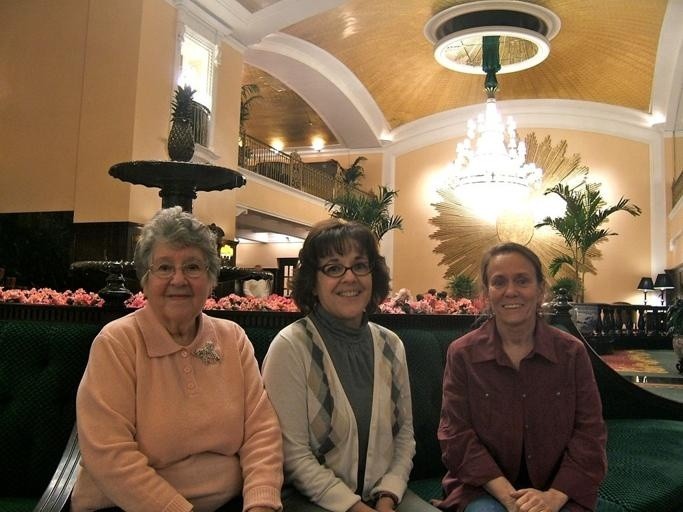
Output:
xmin=71 ymin=205 xmax=285 ymax=512
xmin=430 ymin=243 xmax=607 ymax=512
xmin=262 ymin=219 xmax=441 ymax=512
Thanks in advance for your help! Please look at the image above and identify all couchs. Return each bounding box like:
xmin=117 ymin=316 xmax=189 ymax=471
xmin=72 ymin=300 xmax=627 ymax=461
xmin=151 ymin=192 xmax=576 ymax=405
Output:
xmin=2 ymin=307 xmax=682 ymax=511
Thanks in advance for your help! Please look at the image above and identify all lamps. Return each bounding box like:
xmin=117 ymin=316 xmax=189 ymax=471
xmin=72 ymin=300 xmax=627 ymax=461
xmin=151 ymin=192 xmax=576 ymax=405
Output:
xmin=424 ymin=0 xmax=564 ymax=226
xmin=636 ymin=274 xmax=675 ymax=313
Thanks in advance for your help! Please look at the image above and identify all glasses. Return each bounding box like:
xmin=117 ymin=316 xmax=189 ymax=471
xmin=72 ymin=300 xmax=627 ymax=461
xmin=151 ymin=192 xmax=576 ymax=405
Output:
xmin=147 ymin=263 xmax=209 ymax=279
xmin=317 ymin=262 xmax=373 ymax=278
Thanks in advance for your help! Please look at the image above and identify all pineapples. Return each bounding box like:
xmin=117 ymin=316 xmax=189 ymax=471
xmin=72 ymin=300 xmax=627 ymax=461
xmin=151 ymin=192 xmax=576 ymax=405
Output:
xmin=167 ymin=85 xmax=197 ymax=161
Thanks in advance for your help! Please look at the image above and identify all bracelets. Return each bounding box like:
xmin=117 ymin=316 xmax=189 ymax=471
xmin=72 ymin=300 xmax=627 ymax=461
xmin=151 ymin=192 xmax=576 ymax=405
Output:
xmin=372 ymin=493 xmax=398 ymax=510
